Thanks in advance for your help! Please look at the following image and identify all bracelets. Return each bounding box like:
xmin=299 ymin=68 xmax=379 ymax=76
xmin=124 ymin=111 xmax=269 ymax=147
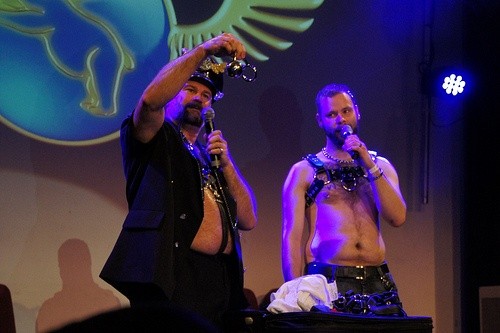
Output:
xmin=364 ymin=165 xmax=384 ymax=182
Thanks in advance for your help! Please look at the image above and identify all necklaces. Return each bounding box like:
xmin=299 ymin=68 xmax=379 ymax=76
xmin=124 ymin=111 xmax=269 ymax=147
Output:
xmin=321 ymin=147 xmax=356 ymax=164
xmin=175 ymin=120 xmax=220 ymax=203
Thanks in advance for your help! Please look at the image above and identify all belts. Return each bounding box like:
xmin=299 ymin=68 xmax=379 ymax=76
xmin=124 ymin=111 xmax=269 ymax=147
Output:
xmin=322 ymin=265 xmax=390 ymax=279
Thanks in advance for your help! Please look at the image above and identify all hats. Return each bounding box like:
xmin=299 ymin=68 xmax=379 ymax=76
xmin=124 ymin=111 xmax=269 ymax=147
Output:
xmin=189 ymin=56 xmax=224 ymax=94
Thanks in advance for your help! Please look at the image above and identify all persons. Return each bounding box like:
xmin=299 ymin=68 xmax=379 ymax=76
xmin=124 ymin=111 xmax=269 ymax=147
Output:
xmin=98 ymin=33 xmax=257 ymax=306
xmin=280 ymin=83 xmax=407 ymax=315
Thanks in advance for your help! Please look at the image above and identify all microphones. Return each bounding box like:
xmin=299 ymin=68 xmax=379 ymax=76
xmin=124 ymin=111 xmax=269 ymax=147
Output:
xmin=341 ymin=125 xmax=359 ymax=160
xmin=201 ymin=107 xmax=221 ymax=169
